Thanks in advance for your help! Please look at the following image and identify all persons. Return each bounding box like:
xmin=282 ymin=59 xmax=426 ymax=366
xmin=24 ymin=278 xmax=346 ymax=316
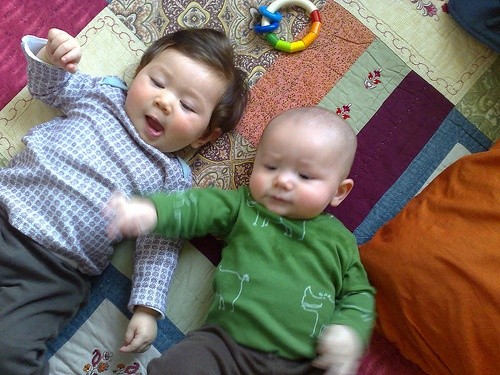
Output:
xmin=103 ymin=106 xmax=377 ymax=375
xmin=0 ymin=27 xmax=251 ymax=375
